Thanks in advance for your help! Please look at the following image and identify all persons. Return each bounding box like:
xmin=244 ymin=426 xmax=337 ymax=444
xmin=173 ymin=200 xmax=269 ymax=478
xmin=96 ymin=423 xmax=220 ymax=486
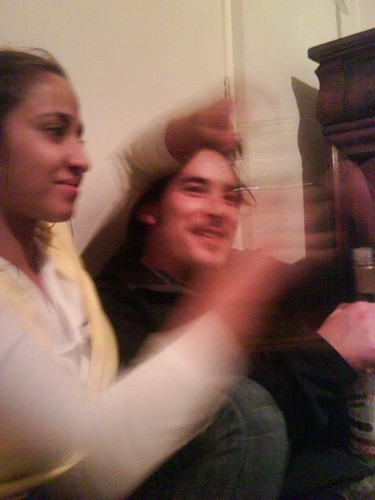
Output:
xmin=1 ymin=44 xmax=339 ymax=500
xmin=95 ymin=128 xmax=375 ymax=500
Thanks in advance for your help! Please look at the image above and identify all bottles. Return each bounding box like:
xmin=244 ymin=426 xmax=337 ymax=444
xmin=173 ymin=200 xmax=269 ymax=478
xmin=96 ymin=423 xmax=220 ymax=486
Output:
xmin=348 ymin=250 xmax=374 ymax=462
